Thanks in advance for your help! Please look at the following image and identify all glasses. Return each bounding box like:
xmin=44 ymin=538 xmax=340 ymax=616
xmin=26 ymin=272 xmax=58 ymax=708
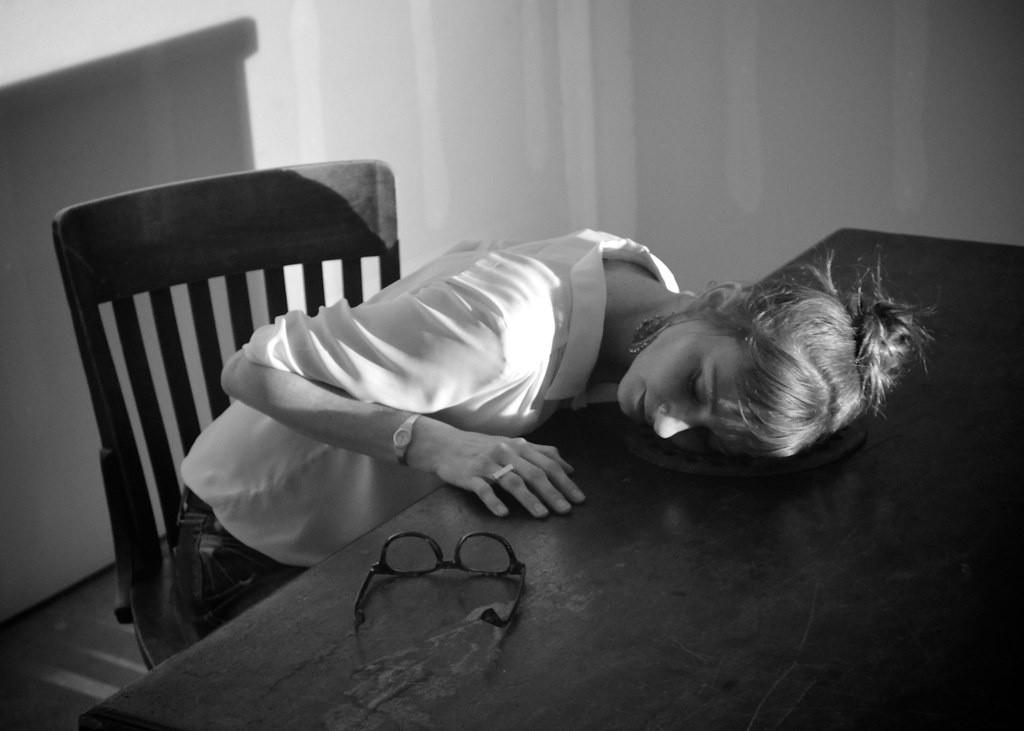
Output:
xmin=354 ymin=531 xmax=526 ymax=628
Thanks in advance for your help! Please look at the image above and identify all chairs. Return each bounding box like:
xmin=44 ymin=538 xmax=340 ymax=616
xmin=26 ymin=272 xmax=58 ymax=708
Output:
xmin=50 ymin=158 xmax=401 ymax=673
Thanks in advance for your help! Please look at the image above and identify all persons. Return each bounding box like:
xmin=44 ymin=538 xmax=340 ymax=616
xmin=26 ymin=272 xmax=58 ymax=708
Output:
xmin=170 ymin=226 xmax=937 ymax=644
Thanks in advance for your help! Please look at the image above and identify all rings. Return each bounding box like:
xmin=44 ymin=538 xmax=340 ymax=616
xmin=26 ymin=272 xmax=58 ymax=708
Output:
xmin=492 ymin=463 xmax=514 ymax=484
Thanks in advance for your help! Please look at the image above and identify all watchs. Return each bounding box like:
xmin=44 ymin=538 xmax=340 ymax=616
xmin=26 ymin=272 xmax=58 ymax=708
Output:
xmin=393 ymin=414 xmax=422 ymax=467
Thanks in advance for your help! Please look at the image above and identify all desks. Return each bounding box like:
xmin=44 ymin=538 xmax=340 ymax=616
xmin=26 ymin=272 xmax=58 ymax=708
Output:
xmin=72 ymin=227 xmax=1024 ymax=731
